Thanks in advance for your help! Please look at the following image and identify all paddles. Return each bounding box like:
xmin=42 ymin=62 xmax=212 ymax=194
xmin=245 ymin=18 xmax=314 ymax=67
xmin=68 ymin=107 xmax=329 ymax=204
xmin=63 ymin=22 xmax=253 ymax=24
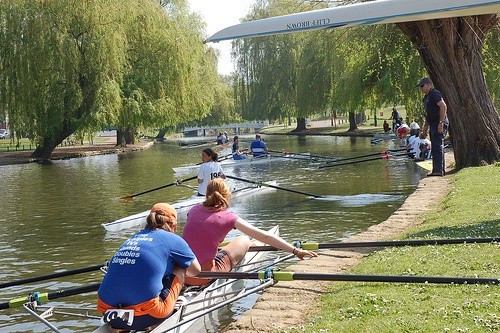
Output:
xmin=219 ymin=236 xmax=500 ymax=252
xmin=0 ymin=282 xmax=103 ymax=310
xmin=189 ymin=269 xmax=500 ymax=286
xmin=245 ymin=152 xmax=322 ymax=162
xmin=0 ymin=262 xmax=111 ymax=288
xmin=225 ymin=174 xmax=325 ymax=197
xmin=327 ymin=148 xmax=415 ymax=164
xmin=197 ymin=148 xmax=248 ymax=165
xmin=120 ymin=176 xmax=197 ymax=200
xmin=318 ymin=152 xmax=417 ymax=169
xmin=268 ymin=149 xmax=336 ymax=159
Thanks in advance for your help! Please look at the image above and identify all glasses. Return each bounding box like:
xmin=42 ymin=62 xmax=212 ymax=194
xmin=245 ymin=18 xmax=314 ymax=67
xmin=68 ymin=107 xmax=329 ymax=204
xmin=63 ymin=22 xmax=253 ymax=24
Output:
xmin=421 ymin=82 xmax=426 ymax=87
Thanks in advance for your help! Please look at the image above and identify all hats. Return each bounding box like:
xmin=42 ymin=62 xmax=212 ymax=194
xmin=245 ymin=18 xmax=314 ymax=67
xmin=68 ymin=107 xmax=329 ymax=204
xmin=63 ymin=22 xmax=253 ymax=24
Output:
xmin=151 ymin=202 xmax=177 ymax=220
xmin=416 ymin=78 xmax=432 ymax=87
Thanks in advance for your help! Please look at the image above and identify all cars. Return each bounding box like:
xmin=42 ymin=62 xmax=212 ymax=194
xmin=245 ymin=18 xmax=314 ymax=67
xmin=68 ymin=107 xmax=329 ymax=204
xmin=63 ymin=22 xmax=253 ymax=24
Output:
xmin=0 ymin=129 xmax=10 ymax=139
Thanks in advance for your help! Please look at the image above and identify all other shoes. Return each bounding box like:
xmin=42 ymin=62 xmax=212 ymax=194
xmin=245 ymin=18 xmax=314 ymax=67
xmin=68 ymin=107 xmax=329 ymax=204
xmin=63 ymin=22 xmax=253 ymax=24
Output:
xmin=427 ymin=172 xmax=444 ymax=176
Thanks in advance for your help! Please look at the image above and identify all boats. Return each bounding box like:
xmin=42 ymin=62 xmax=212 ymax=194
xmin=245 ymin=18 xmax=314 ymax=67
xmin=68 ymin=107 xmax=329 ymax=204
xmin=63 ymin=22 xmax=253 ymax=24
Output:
xmin=88 ymin=224 xmax=280 ymax=333
xmin=100 ymin=180 xmax=277 ymax=233
xmin=171 ymin=152 xmax=311 ymax=173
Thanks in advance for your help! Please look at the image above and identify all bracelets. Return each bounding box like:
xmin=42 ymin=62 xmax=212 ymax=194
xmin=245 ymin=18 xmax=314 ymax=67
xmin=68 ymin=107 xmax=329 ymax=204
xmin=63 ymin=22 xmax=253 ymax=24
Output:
xmin=438 ymin=121 xmax=444 ymax=122
xmin=292 ymin=248 xmax=299 ymax=255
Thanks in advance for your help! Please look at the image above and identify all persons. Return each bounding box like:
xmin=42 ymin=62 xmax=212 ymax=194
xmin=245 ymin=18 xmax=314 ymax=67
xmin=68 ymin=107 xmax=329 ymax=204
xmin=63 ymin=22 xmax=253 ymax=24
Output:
xmin=96 ymin=202 xmax=201 ymax=330
xmin=217 ymin=131 xmax=229 ymax=145
xmin=196 ymin=147 xmax=226 ymax=197
xmin=383 ymin=116 xmax=433 ymax=161
xmin=388 ymin=108 xmax=399 ymax=131
xmin=178 ymin=176 xmax=319 ymax=280
xmin=250 ymin=135 xmax=268 ymax=158
xmin=416 ymin=77 xmax=449 ymax=176
xmin=231 ymin=136 xmax=247 ymax=160
